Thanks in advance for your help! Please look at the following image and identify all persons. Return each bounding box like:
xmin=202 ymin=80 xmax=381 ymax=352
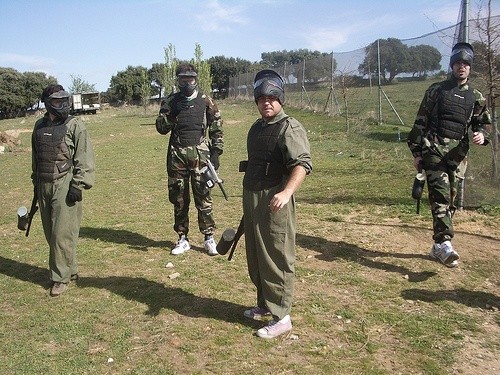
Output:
xmin=155 ymin=64 xmax=225 ymax=255
xmin=239 ymin=70 xmax=313 ymax=339
xmin=31 ymin=85 xmax=94 ymax=298
xmin=407 ymin=42 xmax=493 ymax=264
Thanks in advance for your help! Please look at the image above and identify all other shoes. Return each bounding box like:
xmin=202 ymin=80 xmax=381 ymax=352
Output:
xmin=51 ymin=274 xmax=79 ymax=297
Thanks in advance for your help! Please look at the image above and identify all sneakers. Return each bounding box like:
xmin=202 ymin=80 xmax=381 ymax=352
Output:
xmin=243 ymin=305 xmax=272 ymax=317
xmin=204 ymin=239 xmax=219 ymax=255
xmin=441 ymin=240 xmax=460 ymax=264
xmin=257 ymin=319 xmax=293 ymax=340
xmin=429 ymin=243 xmax=458 ymax=268
xmin=171 ymin=239 xmax=191 ymax=254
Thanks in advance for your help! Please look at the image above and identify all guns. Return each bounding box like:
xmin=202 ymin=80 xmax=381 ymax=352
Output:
xmin=412 ymin=161 xmax=425 ymax=214
xmin=17 ymin=189 xmax=39 ymax=236
xmin=189 ymin=146 xmax=229 ymax=204
xmin=216 ymin=215 xmax=245 ymax=261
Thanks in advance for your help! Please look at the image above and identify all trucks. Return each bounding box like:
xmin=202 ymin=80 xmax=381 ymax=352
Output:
xmin=72 ymin=91 xmax=101 ymax=114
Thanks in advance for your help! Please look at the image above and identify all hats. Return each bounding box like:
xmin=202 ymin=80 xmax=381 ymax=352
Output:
xmin=449 ymin=42 xmax=474 ymax=69
xmin=254 ymin=69 xmax=286 ymax=105
xmin=176 ymin=63 xmax=198 ymax=77
